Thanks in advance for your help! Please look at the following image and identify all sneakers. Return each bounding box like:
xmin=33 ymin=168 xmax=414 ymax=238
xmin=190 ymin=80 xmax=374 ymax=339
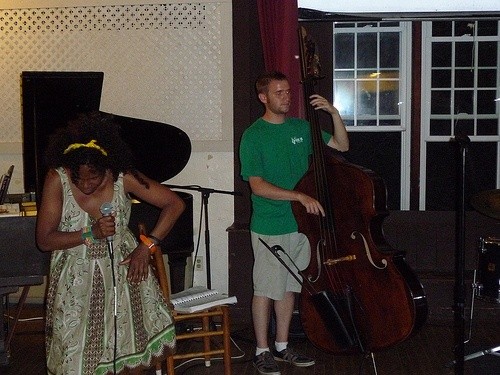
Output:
xmin=252 ymin=351 xmax=281 ymax=375
xmin=273 ymin=349 xmax=316 ymax=367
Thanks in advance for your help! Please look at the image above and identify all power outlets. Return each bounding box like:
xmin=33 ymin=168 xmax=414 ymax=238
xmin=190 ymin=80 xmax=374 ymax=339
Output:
xmin=195 ymin=256 xmax=203 ymax=271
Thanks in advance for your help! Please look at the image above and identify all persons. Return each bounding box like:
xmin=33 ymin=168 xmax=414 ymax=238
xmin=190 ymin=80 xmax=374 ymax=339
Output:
xmin=36 ymin=136 xmax=186 ymax=375
xmin=240 ymin=72 xmax=350 ymax=375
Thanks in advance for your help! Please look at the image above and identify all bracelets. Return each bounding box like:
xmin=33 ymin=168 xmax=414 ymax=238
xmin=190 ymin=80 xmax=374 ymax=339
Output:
xmin=81 ymin=227 xmax=94 ymax=246
xmin=139 ymin=234 xmax=162 ymax=254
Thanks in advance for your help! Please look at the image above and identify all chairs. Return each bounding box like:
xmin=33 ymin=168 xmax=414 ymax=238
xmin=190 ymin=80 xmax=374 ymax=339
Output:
xmin=139 ymin=235 xmax=233 ymax=375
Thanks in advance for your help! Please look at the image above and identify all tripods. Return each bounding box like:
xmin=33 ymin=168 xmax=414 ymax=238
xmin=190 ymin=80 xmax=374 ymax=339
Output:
xmin=161 ymin=184 xmax=255 ymax=345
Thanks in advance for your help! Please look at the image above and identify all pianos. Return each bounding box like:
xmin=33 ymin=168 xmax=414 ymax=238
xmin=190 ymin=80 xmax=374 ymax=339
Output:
xmin=0 ymin=71 xmax=194 ymax=296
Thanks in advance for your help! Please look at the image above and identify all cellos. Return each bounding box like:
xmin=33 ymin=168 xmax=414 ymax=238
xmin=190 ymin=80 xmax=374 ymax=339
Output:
xmin=289 ymin=24 xmax=430 ymax=357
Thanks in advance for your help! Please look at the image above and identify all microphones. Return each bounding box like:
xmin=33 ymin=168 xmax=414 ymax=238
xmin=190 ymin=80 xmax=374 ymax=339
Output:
xmin=99 ymin=202 xmax=115 ymax=259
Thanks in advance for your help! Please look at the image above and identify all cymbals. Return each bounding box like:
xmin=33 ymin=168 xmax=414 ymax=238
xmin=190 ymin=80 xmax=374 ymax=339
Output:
xmin=469 ymin=189 xmax=500 ymax=221
